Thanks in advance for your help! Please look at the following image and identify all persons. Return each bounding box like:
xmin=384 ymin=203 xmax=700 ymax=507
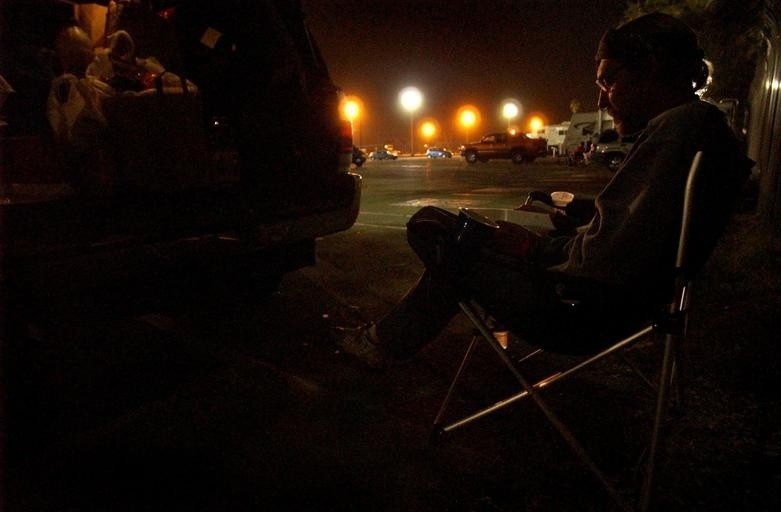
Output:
xmin=321 ymin=13 xmax=755 ymax=391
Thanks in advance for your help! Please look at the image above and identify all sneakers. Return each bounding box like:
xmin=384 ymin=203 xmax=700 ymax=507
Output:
xmin=328 ymin=322 xmax=390 ymax=372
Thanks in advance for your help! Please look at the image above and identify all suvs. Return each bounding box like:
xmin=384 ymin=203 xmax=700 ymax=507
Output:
xmin=0 ymin=2 xmax=362 ymax=319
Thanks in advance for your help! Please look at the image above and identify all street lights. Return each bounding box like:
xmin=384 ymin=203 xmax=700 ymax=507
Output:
xmin=529 ymin=115 xmax=545 ymax=137
xmin=342 ymin=98 xmax=362 ymax=145
xmin=419 ymin=115 xmax=438 ymax=148
xmin=399 ymin=85 xmax=423 ymax=157
xmin=503 ymin=100 xmax=520 ymax=132
xmin=461 ymin=107 xmax=476 ymax=144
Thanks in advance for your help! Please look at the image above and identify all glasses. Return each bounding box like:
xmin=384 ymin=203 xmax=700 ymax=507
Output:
xmin=594 ymin=65 xmax=627 ymax=92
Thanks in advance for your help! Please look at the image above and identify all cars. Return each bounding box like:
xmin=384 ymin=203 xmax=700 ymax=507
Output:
xmin=595 ymin=142 xmax=636 ymax=173
xmin=460 ymin=131 xmax=549 ymax=165
xmin=426 ymin=147 xmax=451 ymax=159
xmin=369 ymin=150 xmax=399 ymax=162
xmin=351 ymin=145 xmax=366 ymax=167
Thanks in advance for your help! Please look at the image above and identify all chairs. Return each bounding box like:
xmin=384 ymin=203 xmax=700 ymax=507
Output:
xmin=427 ymin=147 xmax=710 ymax=512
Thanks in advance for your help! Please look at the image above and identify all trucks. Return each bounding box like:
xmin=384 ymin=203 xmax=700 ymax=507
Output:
xmin=561 ymin=108 xmax=632 ymax=172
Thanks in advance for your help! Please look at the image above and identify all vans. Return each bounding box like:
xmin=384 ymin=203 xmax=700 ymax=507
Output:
xmin=538 ymin=124 xmax=570 ymax=156
